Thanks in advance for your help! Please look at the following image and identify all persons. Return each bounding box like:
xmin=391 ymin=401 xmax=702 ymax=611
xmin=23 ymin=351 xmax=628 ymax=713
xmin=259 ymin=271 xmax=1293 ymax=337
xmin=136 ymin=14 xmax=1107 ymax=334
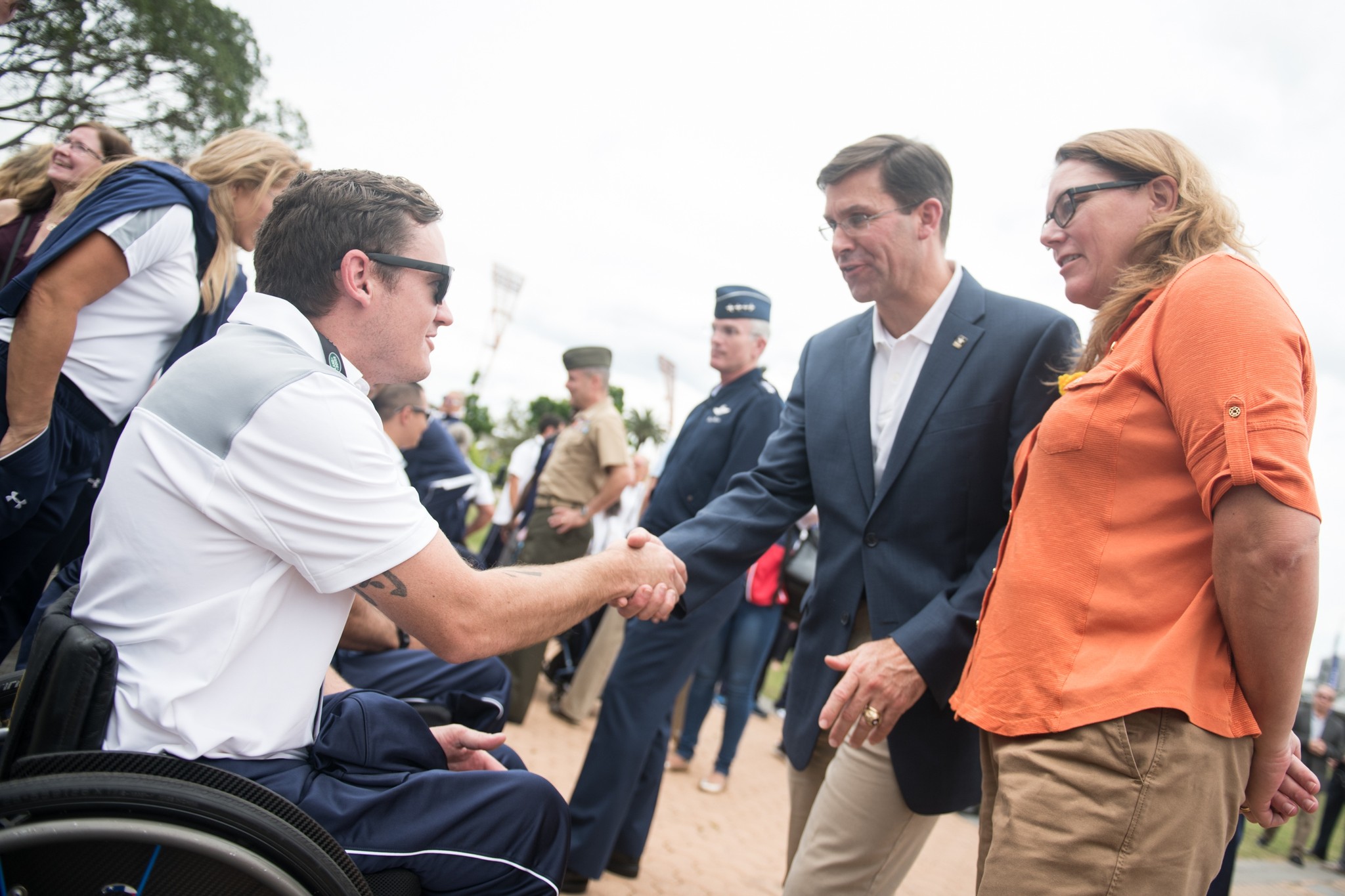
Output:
xmin=0 ymin=116 xmax=1345 ymax=896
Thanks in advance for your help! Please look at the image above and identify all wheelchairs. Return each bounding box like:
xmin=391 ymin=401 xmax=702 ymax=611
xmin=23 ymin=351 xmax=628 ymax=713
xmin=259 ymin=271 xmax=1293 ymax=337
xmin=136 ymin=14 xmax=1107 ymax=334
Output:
xmin=0 ymin=556 xmax=394 ymax=896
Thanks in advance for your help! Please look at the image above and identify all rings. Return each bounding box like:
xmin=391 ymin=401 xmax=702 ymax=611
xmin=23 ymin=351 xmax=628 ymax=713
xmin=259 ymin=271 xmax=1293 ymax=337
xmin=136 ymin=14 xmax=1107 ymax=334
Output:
xmin=1239 ymin=806 xmax=1251 ymax=813
xmin=863 ymin=707 xmax=883 ymax=728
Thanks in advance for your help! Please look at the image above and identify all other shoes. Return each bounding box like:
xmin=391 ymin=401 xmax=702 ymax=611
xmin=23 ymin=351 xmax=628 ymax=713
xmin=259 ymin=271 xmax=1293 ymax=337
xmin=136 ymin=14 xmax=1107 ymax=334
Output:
xmin=559 ymin=871 xmax=586 ymax=893
xmin=1256 ymin=837 xmax=1267 ymax=847
xmin=1304 ymin=850 xmax=1323 ymax=862
xmin=718 ymin=694 xmax=725 ymax=706
xmin=1323 ymin=859 xmax=1343 ymax=872
xmin=665 ymin=756 xmax=692 ymax=772
xmin=604 ymin=855 xmax=640 ymax=879
xmin=699 ymin=774 xmax=729 ymax=792
xmin=1291 ymin=855 xmax=1303 ymax=866
xmin=750 ymin=704 xmax=768 ymax=717
xmin=550 ymin=693 xmax=580 ymax=724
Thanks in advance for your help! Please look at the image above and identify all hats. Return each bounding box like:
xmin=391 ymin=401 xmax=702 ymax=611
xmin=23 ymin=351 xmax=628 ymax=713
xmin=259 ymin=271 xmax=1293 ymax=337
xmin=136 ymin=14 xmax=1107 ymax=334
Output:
xmin=714 ymin=287 xmax=773 ymax=321
xmin=563 ymin=348 xmax=613 ymax=369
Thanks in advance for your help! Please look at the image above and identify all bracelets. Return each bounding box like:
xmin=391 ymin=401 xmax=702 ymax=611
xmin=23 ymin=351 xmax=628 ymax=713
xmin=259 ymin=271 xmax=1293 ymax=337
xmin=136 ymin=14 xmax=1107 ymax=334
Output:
xmin=395 ymin=623 xmax=410 ymax=650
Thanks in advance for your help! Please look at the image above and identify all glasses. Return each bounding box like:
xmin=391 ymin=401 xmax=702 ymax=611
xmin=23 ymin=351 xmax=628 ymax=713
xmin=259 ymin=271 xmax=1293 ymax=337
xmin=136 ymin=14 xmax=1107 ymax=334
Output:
xmin=818 ymin=200 xmax=923 ymax=242
xmin=330 ymin=253 xmax=455 ymax=306
xmin=1315 ymin=692 xmax=1336 ymax=703
xmin=1044 ymin=178 xmax=1149 ymax=252
xmin=55 ymin=135 xmax=107 ymax=166
xmin=392 ymin=405 xmax=432 ymax=421
